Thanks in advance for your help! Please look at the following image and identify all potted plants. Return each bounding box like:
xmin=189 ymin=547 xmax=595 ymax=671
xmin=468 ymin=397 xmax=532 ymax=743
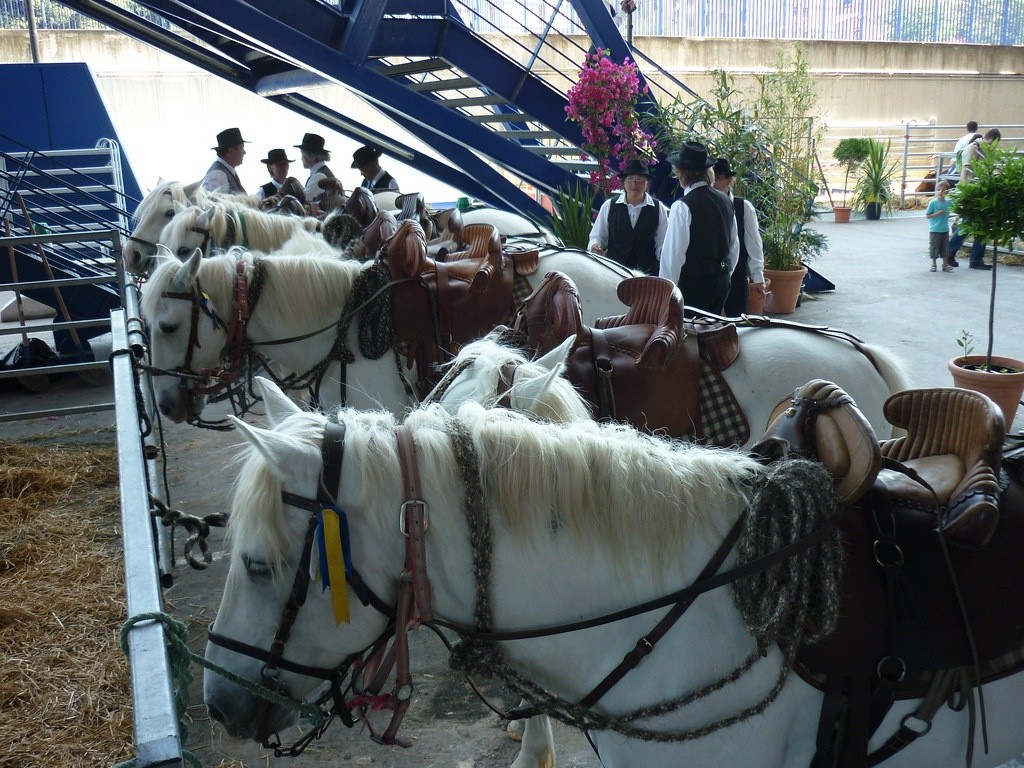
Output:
xmin=832 ymin=137 xmax=871 ymax=223
xmin=632 ymin=49 xmax=830 ymax=316
xmin=943 ymin=137 xmax=1024 ymax=433
xmin=853 ymin=138 xmax=902 ymax=220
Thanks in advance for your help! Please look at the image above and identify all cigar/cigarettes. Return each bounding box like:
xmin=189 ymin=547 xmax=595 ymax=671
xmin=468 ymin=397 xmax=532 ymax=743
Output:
xmin=766 ymin=292 xmax=771 ymax=295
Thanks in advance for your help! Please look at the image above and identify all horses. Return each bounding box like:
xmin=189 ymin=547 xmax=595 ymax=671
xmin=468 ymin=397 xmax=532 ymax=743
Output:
xmin=195 ymin=374 xmax=1024 ymax=767
xmin=391 ymin=317 xmax=910 ymax=768
xmin=137 ymin=245 xmax=652 ymax=425
xmin=158 ymin=202 xmax=562 ymax=262
xmin=121 ymin=178 xmax=405 ymax=271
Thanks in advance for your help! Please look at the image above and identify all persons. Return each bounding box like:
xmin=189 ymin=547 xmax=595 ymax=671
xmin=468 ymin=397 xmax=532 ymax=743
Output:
xmin=926 ymin=182 xmax=954 ymax=272
xmin=948 ymin=121 xmax=984 ymax=173
xmin=253 ymin=149 xmax=296 ymax=204
xmin=657 ymin=142 xmax=741 ymax=315
xmin=587 ymin=160 xmax=670 ymax=275
xmin=203 ymin=127 xmax=253 ymax=197
xmin=351 ymin=147 xmax=398 ymax=192
xmin=711 ymin=158 xmax=767 ymax=319
xmin=293 ymin=134 xmax=341 ymax=203
xmin=947 ymin=129 xmax=1002 ymax=270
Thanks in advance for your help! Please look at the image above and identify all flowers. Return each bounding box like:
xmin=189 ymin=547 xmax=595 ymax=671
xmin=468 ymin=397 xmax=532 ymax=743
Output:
xmin=565 ymin=47 xmax=658 ymax=195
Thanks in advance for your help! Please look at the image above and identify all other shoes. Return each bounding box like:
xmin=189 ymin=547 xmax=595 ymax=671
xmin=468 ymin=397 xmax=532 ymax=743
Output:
xmin=930 ymin=264 xmax=937 ymax=272
xmin=942 ymin=264 xmax=954 ymax=272
xmin=969 ymin=262 xmax=992 ymax=269
xmin=947 ymin=256 xmax=958 ymax=267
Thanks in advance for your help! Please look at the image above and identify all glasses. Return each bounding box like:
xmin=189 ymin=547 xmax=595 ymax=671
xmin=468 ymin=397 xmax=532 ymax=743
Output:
xmin=625 ymin=177 xmax=647 ymax=182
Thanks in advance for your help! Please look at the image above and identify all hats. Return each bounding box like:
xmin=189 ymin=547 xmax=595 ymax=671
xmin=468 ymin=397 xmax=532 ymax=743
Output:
xmin=211 ymin=128 xmax=252 ymax=150
xmin=351 ymin=146 xmax=381 ymax=168
xmin=293 ymin=133 xmax=329 ymax=152
xmin=616 ymin=159 xmax=656 ymax=179
xmin=261 ymin=149 xmax=296 ymax=163
xmin=713 ymin=158 xmax=737 ymax=175
xmin=666 ymin=142 xmax=718 ymax=169
